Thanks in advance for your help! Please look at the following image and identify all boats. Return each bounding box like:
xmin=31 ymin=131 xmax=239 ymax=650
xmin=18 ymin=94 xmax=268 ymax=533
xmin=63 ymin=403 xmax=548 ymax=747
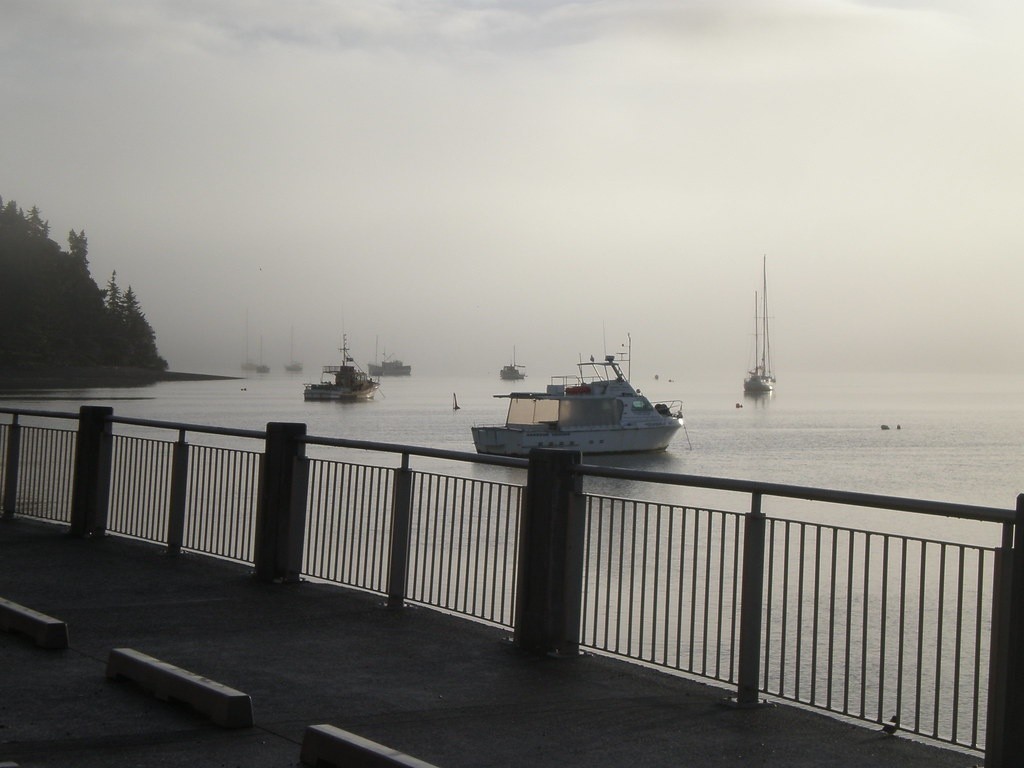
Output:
xmin=500 ymin=344 xmax=527 ymax=381
xmin=368 ymin=334 xmax=412 ymax=376
xmin=303 ymin=334 xmax=381 ymax=400
xmin=470 ymin=331 xmax=686 ymax=459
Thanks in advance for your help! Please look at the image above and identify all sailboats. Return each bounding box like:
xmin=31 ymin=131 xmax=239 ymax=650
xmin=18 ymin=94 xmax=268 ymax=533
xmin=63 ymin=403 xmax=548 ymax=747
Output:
xmin=744 ymin=254 xmax=775 ymax=392
